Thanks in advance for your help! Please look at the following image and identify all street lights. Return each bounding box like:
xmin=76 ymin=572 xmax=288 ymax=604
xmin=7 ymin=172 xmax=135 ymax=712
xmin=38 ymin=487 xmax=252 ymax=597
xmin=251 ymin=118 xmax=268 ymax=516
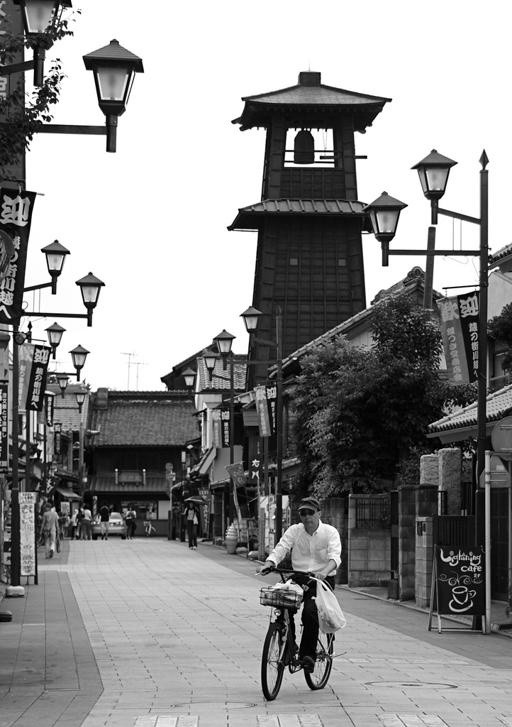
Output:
xmin=184 ymin=303 xmax=286 ymax=565
xmin=363 ymin=144 xmax=491 ymax=638
xmin=1 ymin=237 xmax=108 ymax=602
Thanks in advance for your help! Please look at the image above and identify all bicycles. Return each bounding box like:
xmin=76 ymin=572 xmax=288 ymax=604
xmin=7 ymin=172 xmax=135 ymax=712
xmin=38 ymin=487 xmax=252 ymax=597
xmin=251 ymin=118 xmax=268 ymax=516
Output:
xmin=254 ymin=565 xmax=336 ymax=702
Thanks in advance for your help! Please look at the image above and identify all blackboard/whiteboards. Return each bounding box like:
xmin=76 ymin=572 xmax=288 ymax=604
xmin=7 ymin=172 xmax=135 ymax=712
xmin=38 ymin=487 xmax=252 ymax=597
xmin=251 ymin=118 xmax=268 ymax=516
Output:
xmin=434 ymin=543 xmax=486 ymax=615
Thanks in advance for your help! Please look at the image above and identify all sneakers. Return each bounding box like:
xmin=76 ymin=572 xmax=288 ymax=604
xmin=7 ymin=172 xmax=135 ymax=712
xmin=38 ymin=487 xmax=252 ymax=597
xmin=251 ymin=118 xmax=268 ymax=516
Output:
xmin=190 ymin=546 xmax=196 ymax=550
xmin=49 ymin=550 xmax=53 ymax=558
xmin=303 ymin=656 xmax=314 ymax=673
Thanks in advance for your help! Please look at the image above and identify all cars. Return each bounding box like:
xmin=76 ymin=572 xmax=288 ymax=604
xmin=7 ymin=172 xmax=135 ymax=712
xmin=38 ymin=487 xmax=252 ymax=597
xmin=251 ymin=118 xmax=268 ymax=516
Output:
xmin=92 ymin=511 xmax=128 ymax=539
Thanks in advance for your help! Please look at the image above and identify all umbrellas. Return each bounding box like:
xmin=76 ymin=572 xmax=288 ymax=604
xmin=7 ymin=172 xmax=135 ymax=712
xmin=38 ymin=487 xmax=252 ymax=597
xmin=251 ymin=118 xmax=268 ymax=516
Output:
xmin=183 ymin=493 xmax=208 ymax=504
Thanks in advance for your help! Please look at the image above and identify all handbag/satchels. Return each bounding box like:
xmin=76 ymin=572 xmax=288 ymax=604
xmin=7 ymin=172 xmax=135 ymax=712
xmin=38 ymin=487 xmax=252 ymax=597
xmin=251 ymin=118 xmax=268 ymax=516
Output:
xmin=42 ymin=530 xmax=51 ymax=538
xmin=56 ymin=540 xmax=61 ymax=552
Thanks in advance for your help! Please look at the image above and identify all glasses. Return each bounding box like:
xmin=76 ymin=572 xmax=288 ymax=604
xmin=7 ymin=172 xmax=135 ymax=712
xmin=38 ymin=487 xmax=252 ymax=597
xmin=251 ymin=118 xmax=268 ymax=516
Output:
xmin=300 ymin=509 xmax=315 ymax=516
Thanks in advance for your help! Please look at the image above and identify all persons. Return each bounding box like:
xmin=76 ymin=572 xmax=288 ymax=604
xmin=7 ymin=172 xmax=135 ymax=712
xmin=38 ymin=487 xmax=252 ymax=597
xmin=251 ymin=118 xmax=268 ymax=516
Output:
xmin=257 ymin=494 xmax=343 ymax=673
xmin=40 ymin=499 xmax=202 ymax=558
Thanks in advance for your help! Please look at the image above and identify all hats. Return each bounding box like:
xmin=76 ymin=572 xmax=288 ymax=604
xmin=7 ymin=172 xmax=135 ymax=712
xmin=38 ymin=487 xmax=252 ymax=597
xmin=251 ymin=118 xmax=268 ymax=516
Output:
xmin=297 ymin=497 xmax=321 ymax=512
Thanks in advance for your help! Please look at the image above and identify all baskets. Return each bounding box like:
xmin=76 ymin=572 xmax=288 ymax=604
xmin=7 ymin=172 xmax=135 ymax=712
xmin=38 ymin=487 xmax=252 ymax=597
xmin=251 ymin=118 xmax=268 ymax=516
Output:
xmin=260 ymin=585 xmax=302 ymax=608
